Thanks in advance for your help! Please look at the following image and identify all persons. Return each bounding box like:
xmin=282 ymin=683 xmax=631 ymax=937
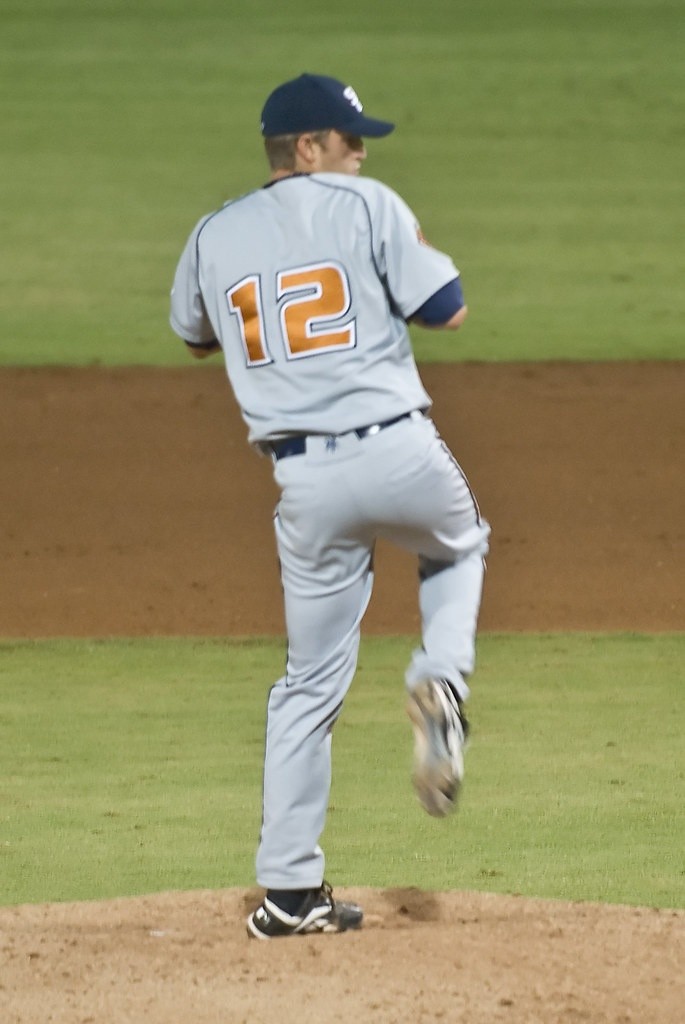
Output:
xmin=171 ymin=71 xmax=493 ymax=939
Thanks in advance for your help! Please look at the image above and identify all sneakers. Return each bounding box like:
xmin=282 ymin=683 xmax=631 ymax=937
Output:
xmin=248 ymin=882 xmax=363 ymax=940
xmin=406 ymin=675 xmax=466 ymax=819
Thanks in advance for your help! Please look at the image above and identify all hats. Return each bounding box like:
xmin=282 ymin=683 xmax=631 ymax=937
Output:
xmin=261 ymin=72 xmax=395 ymax=138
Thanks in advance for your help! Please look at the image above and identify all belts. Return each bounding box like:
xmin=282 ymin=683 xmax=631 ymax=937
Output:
xmin=271 ymin=409 xmax=425 ymax=461
